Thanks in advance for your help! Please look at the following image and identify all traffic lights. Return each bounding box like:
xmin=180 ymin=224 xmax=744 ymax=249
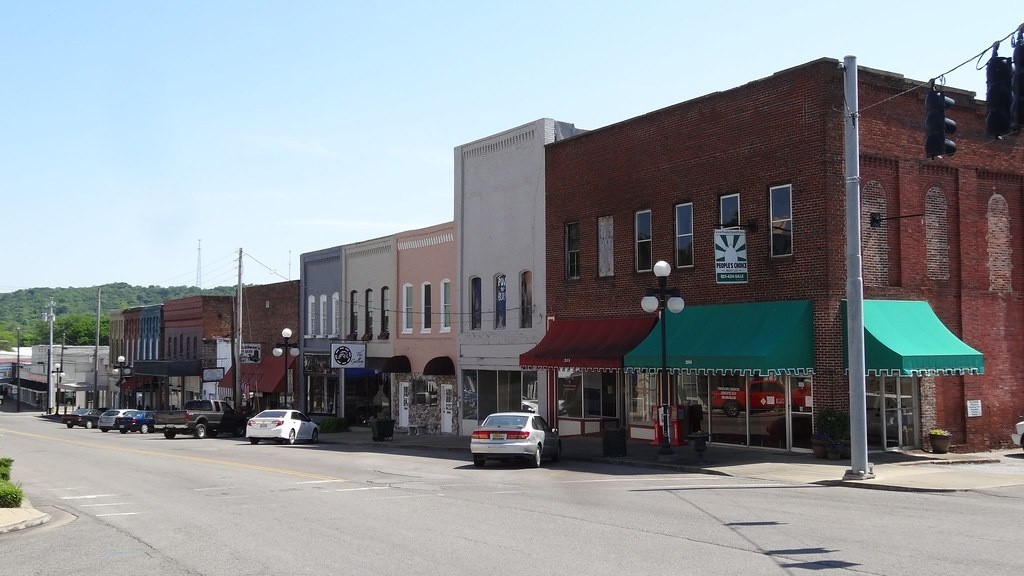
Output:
xmin=925 ymin=89 xmax=957 ymax=158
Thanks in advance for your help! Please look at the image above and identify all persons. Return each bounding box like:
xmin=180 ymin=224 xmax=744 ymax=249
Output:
xmin=36 ymin=399 xmax=40 ymax=408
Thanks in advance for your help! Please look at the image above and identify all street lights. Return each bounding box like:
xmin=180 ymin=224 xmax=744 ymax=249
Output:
xmin=272 ymin=328 xmax=299 ymax=409
xmin=640 ymin=261 xmax=685 ymax=456
xmin=111 ymin=356 xmax=131 ymax=409
xmin=41 ymin=296 xmax=56 ymax=416
xmin=51 ymin=363 xmax=65 ymax=415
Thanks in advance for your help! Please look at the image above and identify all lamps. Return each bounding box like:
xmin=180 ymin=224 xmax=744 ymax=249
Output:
xmin=871 ymin=212 xmax=926 ymax=227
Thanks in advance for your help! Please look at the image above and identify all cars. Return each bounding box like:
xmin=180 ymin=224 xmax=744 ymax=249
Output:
xmin=61 ymin=409 xmax=102 ymax=429
xmin=521 ymin=394 xmax=539 ymax=414
xmin=558 ymin=400 xmax=567 ymax=415
xmin=245 ymin=409 xmax=320 ymax=446
xmin=98 ymin=409 xmax=139 ymax=432
xmin=470 ymin=412 xmax=562 ymax=468
xmin=792 ymin=385 xmax=811 ymax=396
xmin=113 ymin=410 xmax=156 ymax=434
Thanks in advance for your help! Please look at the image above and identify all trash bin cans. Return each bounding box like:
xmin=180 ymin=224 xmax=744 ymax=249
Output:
xmin=601 ymin=430 xmax=628 ymax=458
xmin=368 ymin=419 xmax=395 ymax=441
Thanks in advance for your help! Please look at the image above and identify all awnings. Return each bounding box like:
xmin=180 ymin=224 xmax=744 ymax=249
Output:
xmin=841 ymin=299 xmax=984 ymax=377
xmin=120 ymin=376 xmax=150 ymax=390
xmin=423 ymin=356 xmax=455 ymax=375
xmin=382 ymin=355 xmax=411 ymax=373
xmin=623 ymin=299 xmax=817 ymax=376
xmin=217 ymin=351 xmax=296 ymax=393
xmin=6 ymin=378 xmax=48 ymax=393
xmin=519 ymin=317 xmax=660 ymax=374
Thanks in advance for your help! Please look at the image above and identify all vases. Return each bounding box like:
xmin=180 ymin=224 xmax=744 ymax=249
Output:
xmin=826 ymin=450 xmax=841 ymax=460
xmin=810 ymin=439 xmax=827 ymax=458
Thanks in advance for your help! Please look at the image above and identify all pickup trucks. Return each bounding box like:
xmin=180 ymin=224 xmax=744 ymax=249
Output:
xmin=153 ymin=399 xmax=247 ymax=439
xmin=712 ymin=380 xmax=810 ymax=418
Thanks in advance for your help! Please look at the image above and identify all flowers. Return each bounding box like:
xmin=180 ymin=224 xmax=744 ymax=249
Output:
xmin=814 ymin=433 xmax=830 ymax=440
xmin=823 ymin=441 xmax=843 ymax=450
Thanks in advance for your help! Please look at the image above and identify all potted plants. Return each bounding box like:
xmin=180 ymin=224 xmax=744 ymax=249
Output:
xmin=928 ymin=429 xmax=952 ymax=454
xmin=815 ymin=406 xmax=850 ymax=460
